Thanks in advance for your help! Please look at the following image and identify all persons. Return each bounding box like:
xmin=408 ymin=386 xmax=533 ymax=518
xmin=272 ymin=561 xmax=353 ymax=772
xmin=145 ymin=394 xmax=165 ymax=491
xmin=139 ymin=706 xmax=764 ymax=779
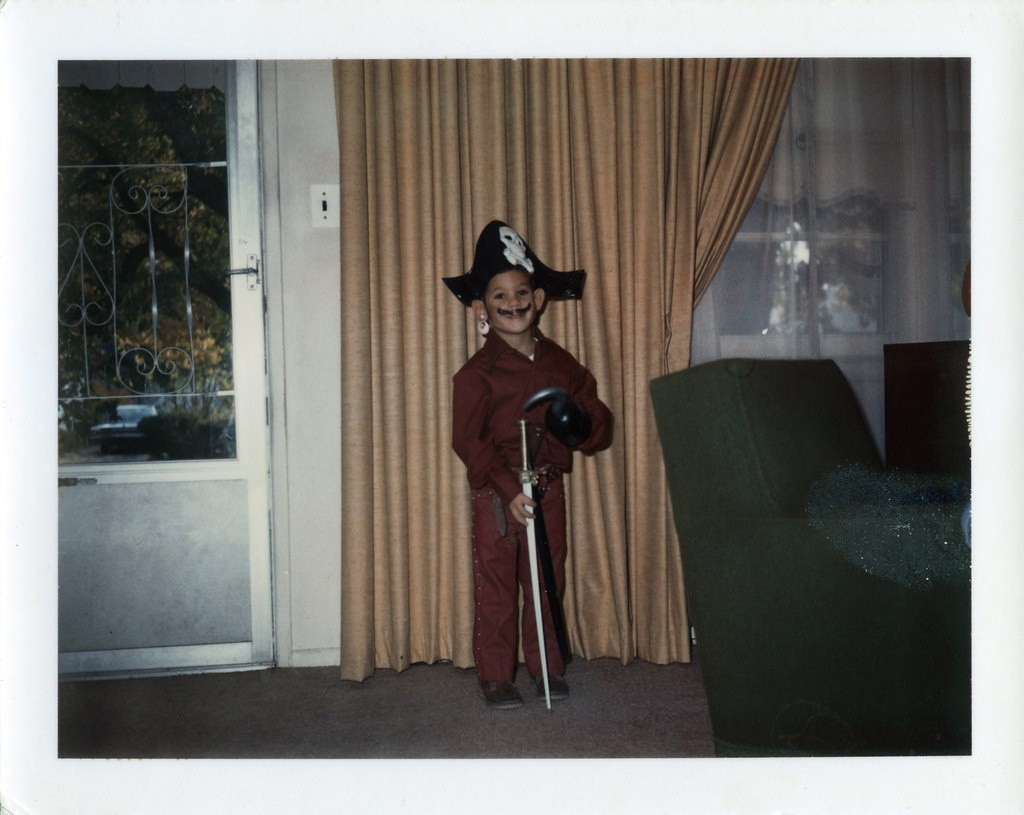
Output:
xmin=441 ymin=219 xmax=614 ymax=709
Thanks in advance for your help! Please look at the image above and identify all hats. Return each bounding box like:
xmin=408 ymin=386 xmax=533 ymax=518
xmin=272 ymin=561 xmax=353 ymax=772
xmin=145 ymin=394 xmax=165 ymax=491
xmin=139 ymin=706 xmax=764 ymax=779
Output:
xmin=441 ymin=220 xmax=587 ymax=306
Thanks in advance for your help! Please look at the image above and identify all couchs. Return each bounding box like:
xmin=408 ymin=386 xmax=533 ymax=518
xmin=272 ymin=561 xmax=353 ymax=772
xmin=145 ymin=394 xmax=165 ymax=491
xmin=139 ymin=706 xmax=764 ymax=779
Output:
xmin=649 ymin=358 xmax=971 ymax=759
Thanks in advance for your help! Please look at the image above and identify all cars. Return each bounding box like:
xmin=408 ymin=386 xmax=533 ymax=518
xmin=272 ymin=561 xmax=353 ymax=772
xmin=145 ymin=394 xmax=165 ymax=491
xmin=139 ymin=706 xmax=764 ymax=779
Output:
xmin=88 ymin=402 xmax=160 ymax=457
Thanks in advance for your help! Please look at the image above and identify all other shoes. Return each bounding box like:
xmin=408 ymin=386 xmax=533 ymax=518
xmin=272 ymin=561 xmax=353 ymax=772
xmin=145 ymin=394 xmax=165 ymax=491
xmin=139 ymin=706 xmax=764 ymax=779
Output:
xmin=477 ymin=679 xmax=523 ymax=709
xmin=531 ymin=673 xmax=568 ymax=700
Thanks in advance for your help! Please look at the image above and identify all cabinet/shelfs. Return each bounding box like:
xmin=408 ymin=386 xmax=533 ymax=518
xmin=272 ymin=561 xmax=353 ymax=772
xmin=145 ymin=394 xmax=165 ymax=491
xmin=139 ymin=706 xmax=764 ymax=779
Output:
xmin=882 ymin=340 xmax=970 ymax=472
